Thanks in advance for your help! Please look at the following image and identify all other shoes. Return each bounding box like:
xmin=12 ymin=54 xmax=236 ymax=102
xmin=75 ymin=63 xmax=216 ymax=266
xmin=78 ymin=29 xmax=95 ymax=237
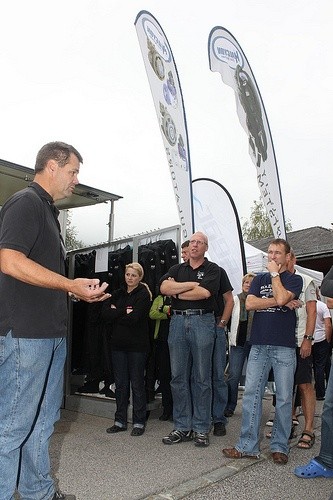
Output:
xmin=213 ymin=422 xmax=226 ymax=436
xmin=224 ymin=409 xmax=234 ymax=418
xmin=159 ymin=415 xmax=173 ymax=423
xmin=265 ymin=419 xmax=274 ymax=427
xmin=222 ymin=447 xmax=260 ymax=459
xmin=106 ymin=424 xmax=127 ymax=433
xmin=131 ymin=427 xmax=145 ymax=436
xmin=52 ymin=491 xmax=65 ymax=500
xmin=273 ymin=452 xmax=288 ymax=465
xmin=292 ymin=417 xmax=299 ymax=425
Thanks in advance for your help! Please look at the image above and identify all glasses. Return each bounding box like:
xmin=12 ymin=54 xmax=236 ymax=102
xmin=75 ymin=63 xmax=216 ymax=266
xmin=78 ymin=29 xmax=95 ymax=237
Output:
xmin=190 ymin=240 xmax=208 ymax=245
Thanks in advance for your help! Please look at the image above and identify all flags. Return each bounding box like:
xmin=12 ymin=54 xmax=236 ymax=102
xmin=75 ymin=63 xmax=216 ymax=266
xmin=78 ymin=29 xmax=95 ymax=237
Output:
xmin=207 ymin=26 xmax=287 ymax=241
xmin=192 ymin=178 xmax=249 ymax=304
xmin=134 ymin=10 xmax=194 ymax=242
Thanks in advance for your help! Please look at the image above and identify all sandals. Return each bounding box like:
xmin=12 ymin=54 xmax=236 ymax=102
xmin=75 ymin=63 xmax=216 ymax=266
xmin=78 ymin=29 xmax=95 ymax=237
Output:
xmin=298 ymin=430 xmax=316 ymax=449
xmin=294 ymin=458 xmax=333 ymax=479
xmin=266 ymin=424 xmax=298 ymax=439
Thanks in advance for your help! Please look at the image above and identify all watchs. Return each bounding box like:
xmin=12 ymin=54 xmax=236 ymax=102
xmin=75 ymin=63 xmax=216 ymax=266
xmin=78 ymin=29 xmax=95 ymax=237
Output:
xmin=269 ymin=271 xmax=280 ymax=278
xmin=69 ymin=292 xmax=79 ymax=303
xmin=302 ymin=334 xmax=315 ymax=341
xmin=220 ymin=319 xmax=228 ymax=325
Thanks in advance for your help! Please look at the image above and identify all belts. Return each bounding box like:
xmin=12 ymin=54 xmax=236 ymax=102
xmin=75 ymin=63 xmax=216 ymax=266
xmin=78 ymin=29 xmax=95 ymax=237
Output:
xmin=171 ymin=309 xmax=213 ymax=316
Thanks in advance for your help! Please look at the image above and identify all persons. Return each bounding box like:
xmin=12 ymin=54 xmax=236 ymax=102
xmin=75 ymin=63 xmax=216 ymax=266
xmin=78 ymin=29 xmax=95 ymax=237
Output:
xmin=107 ymin=233 xmax=333 ymax=478
xmin=0 ymin=141 xmax=111 ymax=500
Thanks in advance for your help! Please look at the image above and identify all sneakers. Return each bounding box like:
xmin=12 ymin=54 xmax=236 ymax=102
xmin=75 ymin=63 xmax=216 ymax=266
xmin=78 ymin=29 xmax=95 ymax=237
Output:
xmin=194 ymin=432 xmax=210 ymax=448
xmin=162 ymin=429 xmax=194 ymax=445
